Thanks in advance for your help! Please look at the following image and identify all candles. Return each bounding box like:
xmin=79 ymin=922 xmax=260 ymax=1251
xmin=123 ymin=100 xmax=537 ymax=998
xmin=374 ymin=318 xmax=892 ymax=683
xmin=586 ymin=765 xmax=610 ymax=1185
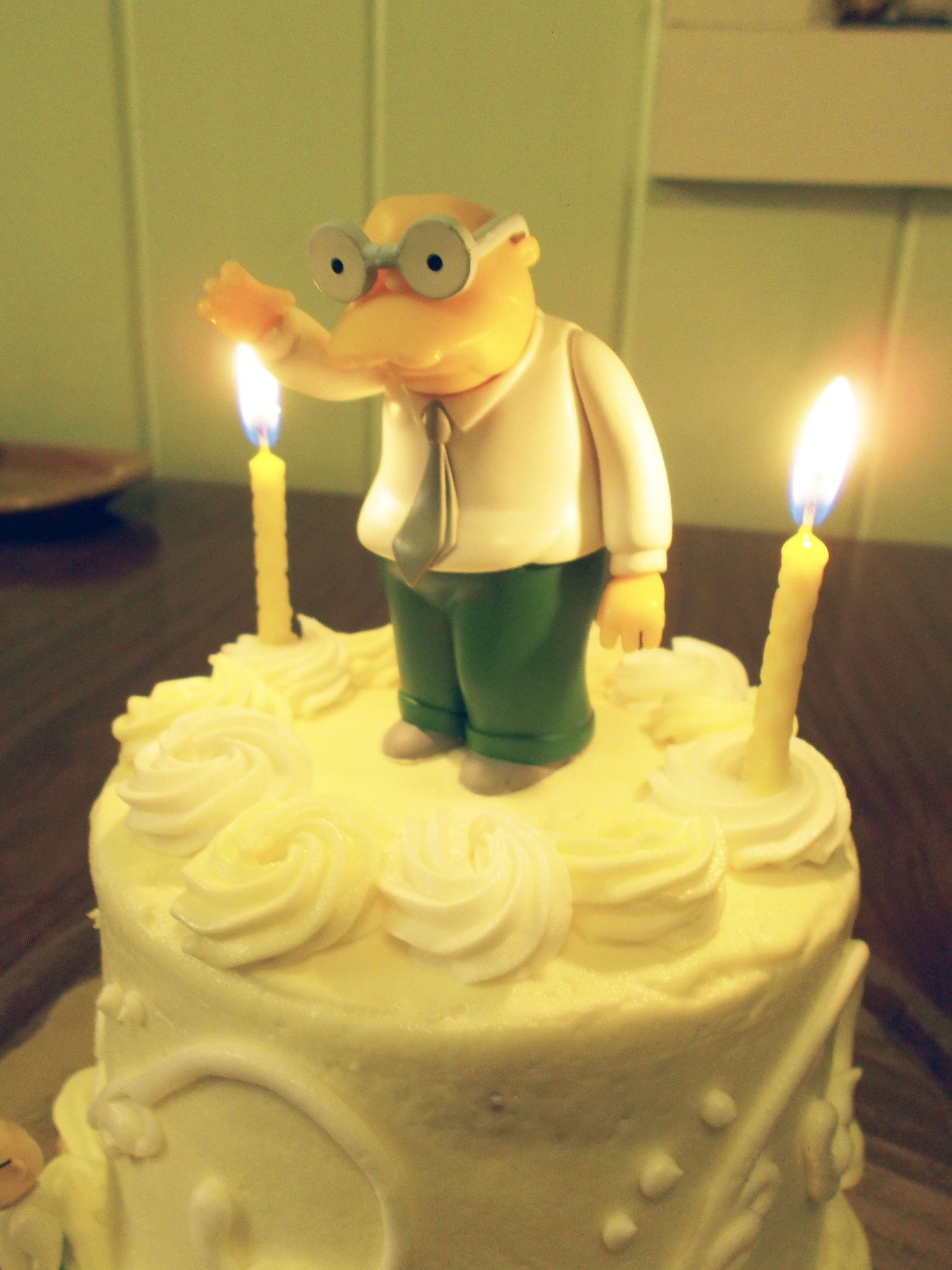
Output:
xmin=737 ymin=371 xmax=866 ymax=787
xmin=232 ymin=345 xmax=297 ymax=648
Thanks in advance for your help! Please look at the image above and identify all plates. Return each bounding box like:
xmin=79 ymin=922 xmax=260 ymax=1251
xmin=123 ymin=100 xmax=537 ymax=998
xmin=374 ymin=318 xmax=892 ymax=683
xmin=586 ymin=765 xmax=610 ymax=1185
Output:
xmin=0 ymin=440 xmax=152 ymax=514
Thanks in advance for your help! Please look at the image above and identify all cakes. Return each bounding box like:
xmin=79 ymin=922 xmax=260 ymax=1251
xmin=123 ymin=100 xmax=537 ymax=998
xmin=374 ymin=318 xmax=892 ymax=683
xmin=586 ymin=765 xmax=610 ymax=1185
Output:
xmin=32 ymin=186 xmax=876 ymax=1270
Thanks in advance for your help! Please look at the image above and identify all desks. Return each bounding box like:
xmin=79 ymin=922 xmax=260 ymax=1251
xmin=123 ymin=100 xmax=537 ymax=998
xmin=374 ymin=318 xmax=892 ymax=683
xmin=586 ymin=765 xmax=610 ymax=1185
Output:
xmin=0 ymin=471 xmax=952 ymax=1270
